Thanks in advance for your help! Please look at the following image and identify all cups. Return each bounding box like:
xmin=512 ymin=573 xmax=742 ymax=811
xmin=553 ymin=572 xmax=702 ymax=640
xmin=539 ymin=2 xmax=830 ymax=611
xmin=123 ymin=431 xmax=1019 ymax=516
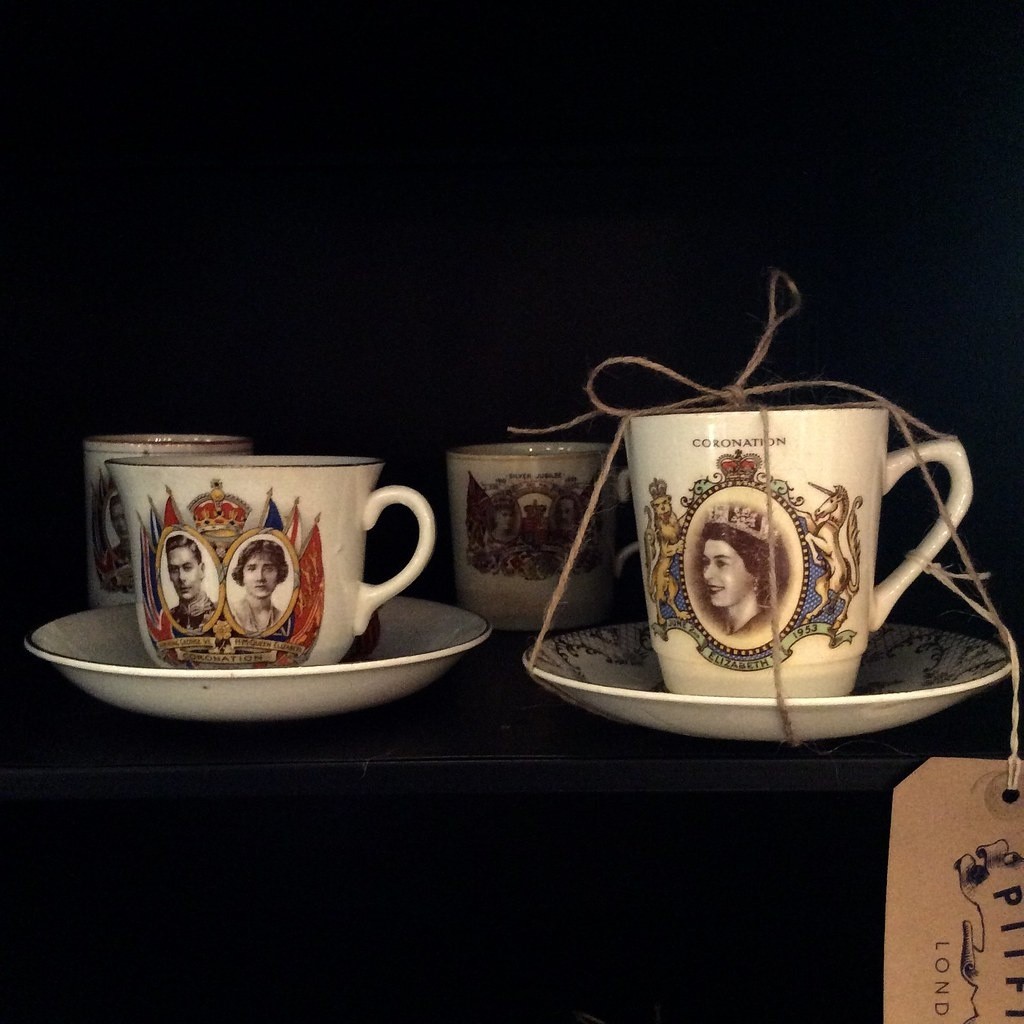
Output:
xmin=623 ymin=407 xmax=973 ymax=700
xmin=447 ymin=441 xmax=644 ymax=632
xmin=103 ymin=452 xmax=436 ymax=671
xmin=81 ymin=432 xmax=258 ymax=610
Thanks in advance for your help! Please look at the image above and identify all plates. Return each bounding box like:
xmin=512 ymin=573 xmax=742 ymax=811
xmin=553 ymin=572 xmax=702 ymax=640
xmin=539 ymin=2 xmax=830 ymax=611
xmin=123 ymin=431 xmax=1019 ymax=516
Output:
xmin=523 ymin=618 xmax=1014 ymax=743
xmin=25 ymin=594 xmax=493 ymax=722
